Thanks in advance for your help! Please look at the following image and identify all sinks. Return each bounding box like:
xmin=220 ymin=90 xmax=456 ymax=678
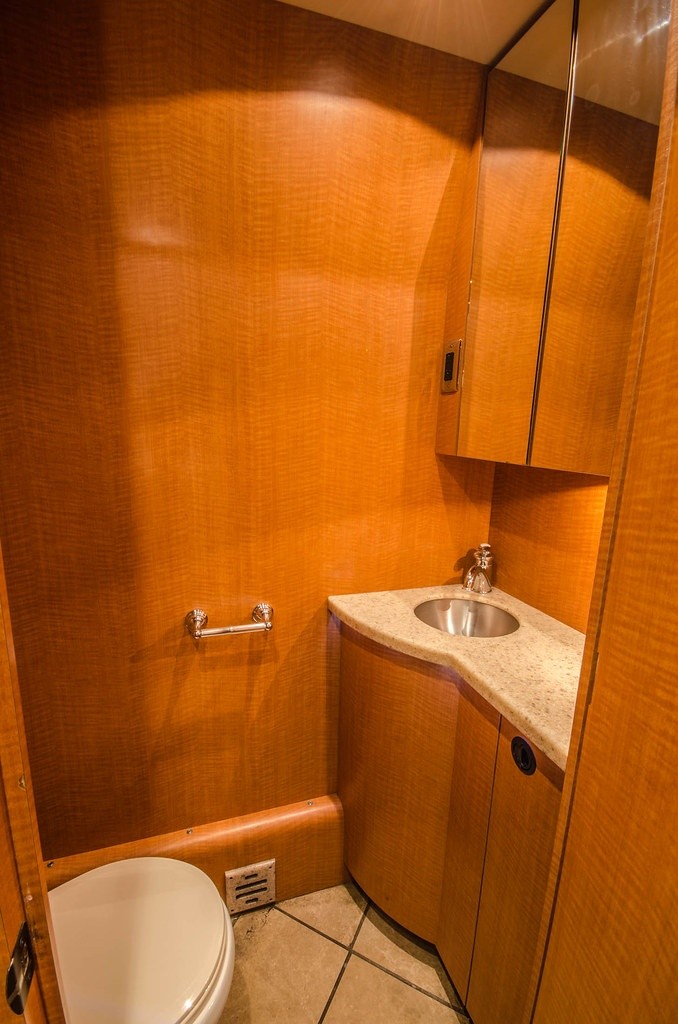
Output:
xmin=411 ymin=593 xmax=521 ymax=638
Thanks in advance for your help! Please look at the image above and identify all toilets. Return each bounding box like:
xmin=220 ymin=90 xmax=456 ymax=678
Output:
xmin=46 ymin=856 xmax=236 ymax=1024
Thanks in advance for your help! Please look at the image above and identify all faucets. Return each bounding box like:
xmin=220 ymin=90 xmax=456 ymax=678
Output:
xmin=463 ymin=543 xmax=493 ymax=595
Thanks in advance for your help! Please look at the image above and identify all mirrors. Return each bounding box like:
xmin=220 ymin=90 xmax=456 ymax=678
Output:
xmin=454 ymin=0 xmax=670 ymax=478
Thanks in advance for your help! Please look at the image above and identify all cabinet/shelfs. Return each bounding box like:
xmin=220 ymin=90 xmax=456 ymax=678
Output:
xmin=465 ymin=715 xmax=565 ymax=1024
